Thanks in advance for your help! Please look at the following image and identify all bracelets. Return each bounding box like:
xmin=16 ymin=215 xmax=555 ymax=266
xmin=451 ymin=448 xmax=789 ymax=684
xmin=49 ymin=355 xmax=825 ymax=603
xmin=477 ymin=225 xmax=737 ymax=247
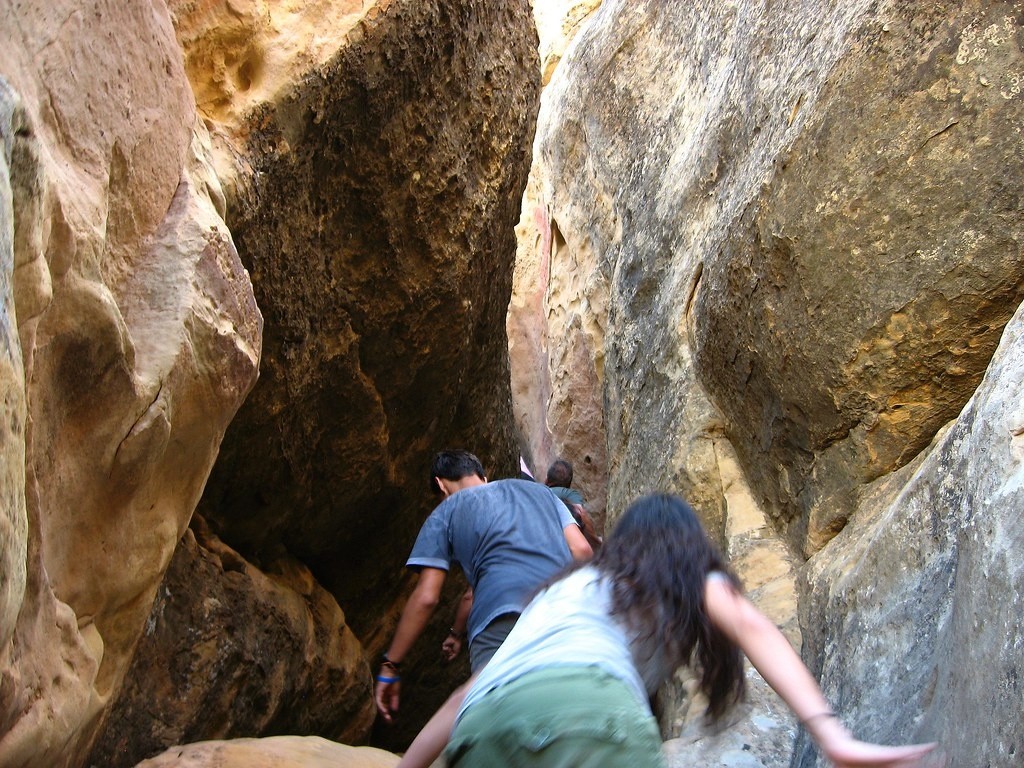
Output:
xmin=382 ymin=655 xmax=405 ymax=672
xmin=800 ymin=711 xmax=837 ymax=725
xmin=376 ymin=676 xmax=399 ymax=683
xmin=449 ymin=628 xmax=463 ymax=639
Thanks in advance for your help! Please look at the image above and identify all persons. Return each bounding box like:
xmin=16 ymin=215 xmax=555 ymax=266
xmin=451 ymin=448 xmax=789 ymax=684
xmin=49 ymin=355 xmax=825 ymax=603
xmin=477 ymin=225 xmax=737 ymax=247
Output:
xmin=374 ymin=449 xmax=594 ymax=723
xmin=543 ymin=459 xmax=602 ymax=545
xmin=396 ymin=492 xmax=939 ymax=768
